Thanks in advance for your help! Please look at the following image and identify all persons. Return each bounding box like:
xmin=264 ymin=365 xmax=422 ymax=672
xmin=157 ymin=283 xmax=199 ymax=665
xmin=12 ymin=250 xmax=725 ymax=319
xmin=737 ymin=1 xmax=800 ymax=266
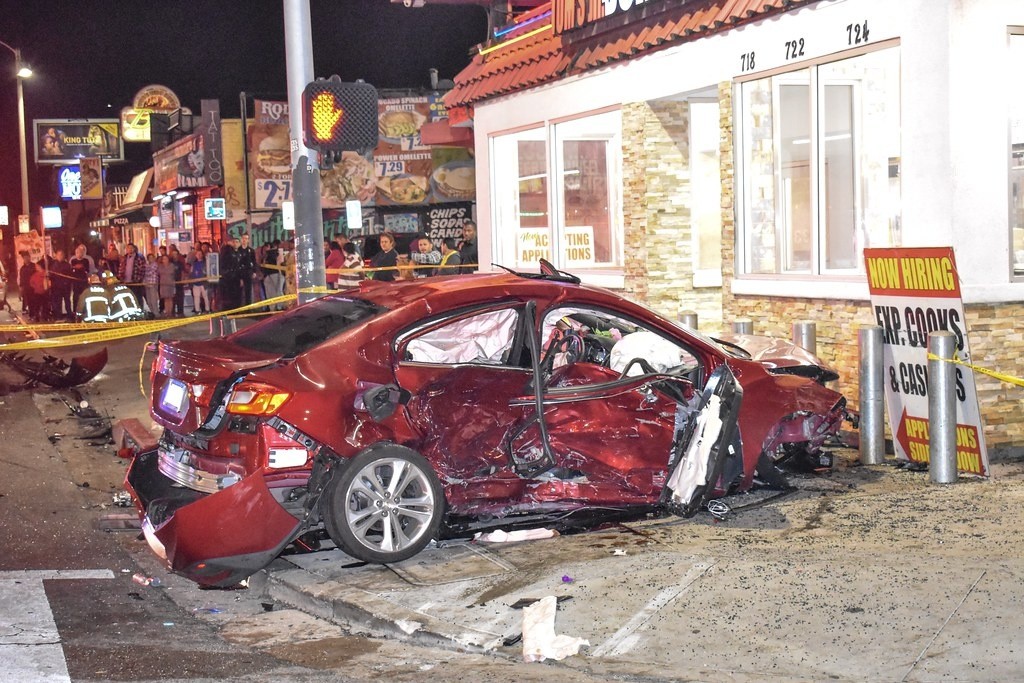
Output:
xmin=12 ymin=220 xmax=477 ymax=325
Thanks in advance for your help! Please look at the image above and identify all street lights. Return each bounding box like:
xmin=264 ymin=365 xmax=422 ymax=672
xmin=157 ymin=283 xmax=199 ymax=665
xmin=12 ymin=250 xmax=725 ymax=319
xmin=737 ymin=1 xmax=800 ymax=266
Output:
xmin=15 ymin=48 xmax=32 ymax=232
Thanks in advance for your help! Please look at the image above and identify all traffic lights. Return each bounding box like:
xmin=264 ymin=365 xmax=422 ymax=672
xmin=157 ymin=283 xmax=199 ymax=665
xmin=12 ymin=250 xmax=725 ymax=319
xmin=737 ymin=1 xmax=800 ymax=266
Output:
xmin=301 ymin=82 xmax=381 ymax=150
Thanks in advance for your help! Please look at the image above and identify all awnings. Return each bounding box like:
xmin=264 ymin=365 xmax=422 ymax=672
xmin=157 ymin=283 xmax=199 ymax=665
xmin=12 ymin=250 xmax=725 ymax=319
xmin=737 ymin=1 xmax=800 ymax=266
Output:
xmin=89 ymin=206 xmax=155 ymax=227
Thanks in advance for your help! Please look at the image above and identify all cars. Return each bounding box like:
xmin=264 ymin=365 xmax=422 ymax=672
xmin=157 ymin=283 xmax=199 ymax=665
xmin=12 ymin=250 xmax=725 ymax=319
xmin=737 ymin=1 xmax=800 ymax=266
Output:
xmin=111 ymin=265 xmax=851 ymax=586
